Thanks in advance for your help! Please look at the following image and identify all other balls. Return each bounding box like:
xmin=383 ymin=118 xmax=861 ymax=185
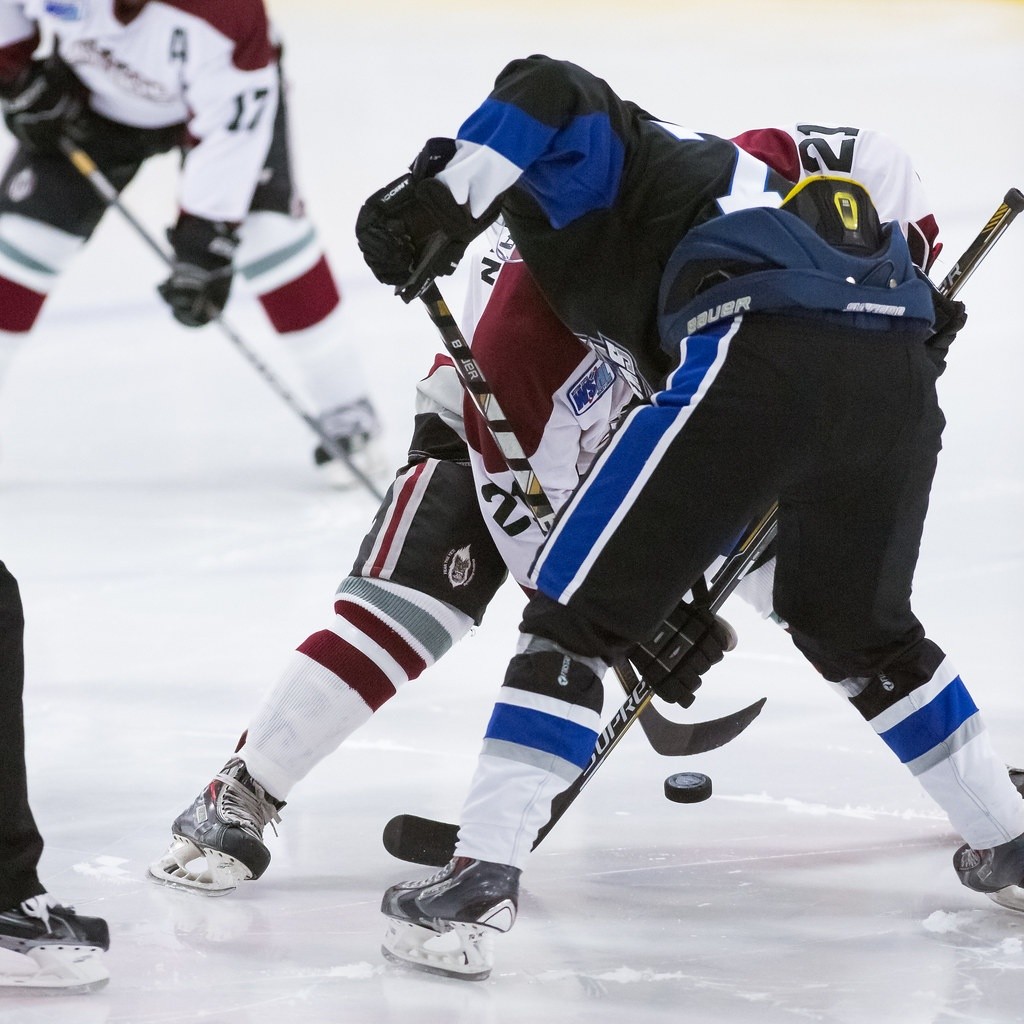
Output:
xmin=664 ymin=772 xmax=711 ymax=804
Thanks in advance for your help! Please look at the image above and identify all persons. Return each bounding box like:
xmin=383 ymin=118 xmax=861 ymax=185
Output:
xmin=0 ymin=0 xmax=377 ymax=468
xmin=170 ymin=56 xmax=1024 ymax=985
xmin=0 ymin=557 xmax=108 ymax=996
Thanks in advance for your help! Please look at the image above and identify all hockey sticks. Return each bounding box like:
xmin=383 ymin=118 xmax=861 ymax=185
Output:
xmin=389 ymin=217 xmax=768 ymax=756
xmin=61 ymin=137 xmax=391 ymax=505
xmin=383 ymin=183 xmax=1024 ymax=873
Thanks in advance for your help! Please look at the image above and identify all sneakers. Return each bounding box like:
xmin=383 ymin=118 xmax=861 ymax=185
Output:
xmin=380 ymin=855 xmax=523 ymax=934
xmin=952 ymin=831 xmax=1024 ymax=894
xmin=0 ymin=890 xmax=110 ymax=955
xmin=171 ymin=756 xmax=288 ymax=881
xmin=313 ymin=397 xmax=380 ymax=465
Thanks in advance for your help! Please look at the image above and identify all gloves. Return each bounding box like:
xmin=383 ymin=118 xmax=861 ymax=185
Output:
xmin=0 ymin=55 xmax=90 ymax=166
xmin=626 ymin=597 xmax=730 ymax=709
xmin=355 ymin=137 xmax=503 ymax=305
xmin=157 ymin=210 xmax=242 ymax=326
